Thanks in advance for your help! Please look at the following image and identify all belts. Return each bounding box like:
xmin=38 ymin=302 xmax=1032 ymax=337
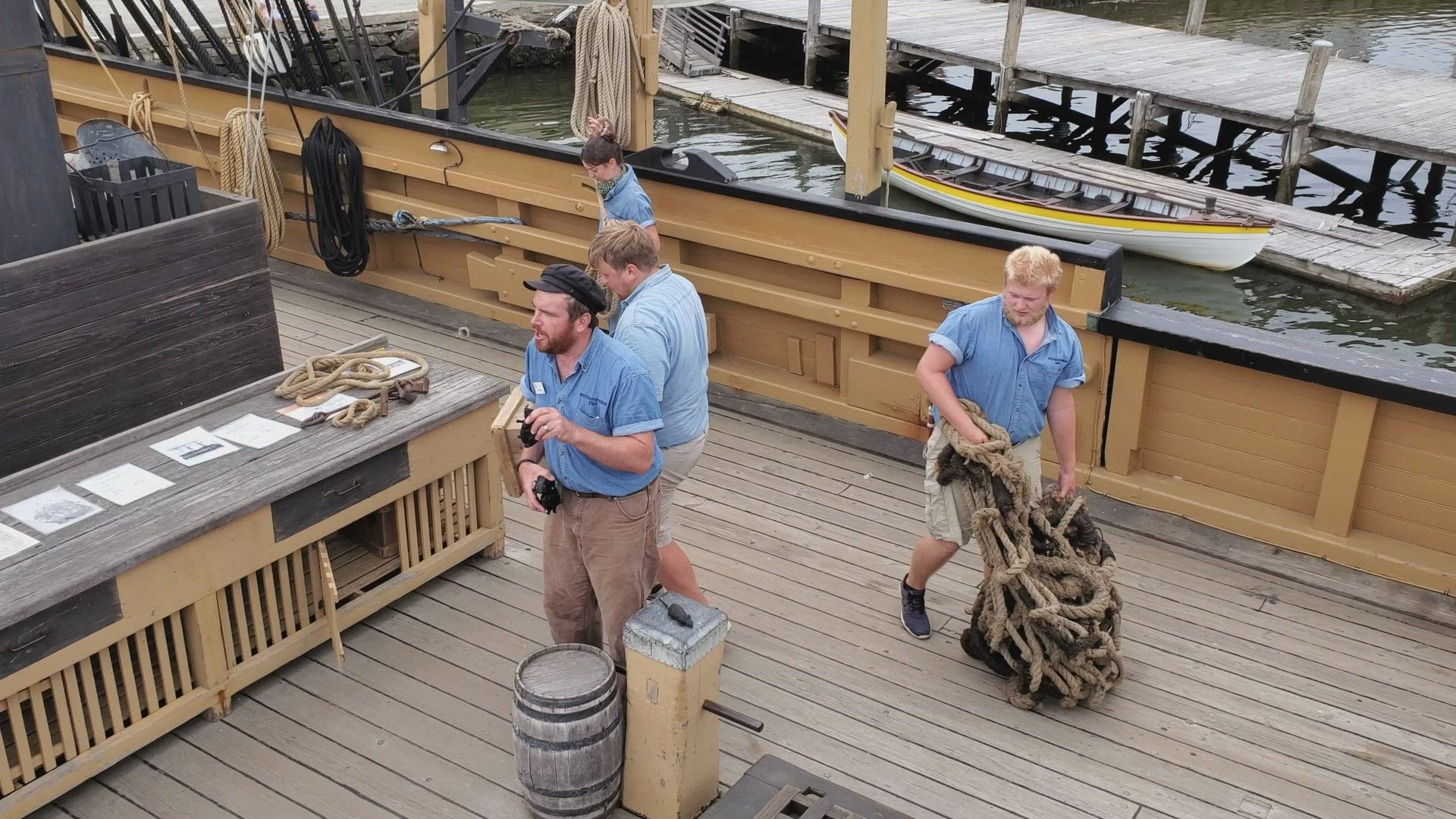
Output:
xmin=561 ymin=472 xmax=660 ymax=498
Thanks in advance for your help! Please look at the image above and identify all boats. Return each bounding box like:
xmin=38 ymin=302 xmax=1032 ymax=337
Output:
xmin=829 ymin=110 xmax=1275 ymax=272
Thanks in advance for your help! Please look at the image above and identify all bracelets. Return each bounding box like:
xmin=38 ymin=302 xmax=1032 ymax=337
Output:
xmin=517 ymin=459 xmax=537 ymax=472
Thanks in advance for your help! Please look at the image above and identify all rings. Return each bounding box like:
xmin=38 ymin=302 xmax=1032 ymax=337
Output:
xmin=544 ymin=425 xmax=548 ymax=432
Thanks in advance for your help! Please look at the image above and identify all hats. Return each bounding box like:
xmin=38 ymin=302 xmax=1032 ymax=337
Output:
xmin=522 ymin=263 xmax=607 ymax=314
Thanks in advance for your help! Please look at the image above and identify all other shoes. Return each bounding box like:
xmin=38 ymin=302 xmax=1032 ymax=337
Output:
xmin=728 ymin=621 xmax=732 ymax=634
xmin=646 ymin=583 xmax=667 ymax=601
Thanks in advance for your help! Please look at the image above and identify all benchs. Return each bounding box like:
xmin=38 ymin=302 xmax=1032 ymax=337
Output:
xmin=925 ymin=164 xmax=980 ymax=182
xmin=1088 ymin=201 xmax=1129 ymax=216
xmin=897 ymin=153 xmax=936 ymax=163
xmin=1044 ymin=189 xmax=1085 ymax=204
xmin=983 ymin=180 xmax=1034 ymax=192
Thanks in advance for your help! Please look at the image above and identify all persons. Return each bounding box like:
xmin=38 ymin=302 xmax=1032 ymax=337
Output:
xmin=588 ymin=220 xmax=731 ymax=632
xmin=308 ymin=4 xmax=319 ymax=28
xmin=899 ymin=245 xmax=1086 ymax=640
xmin=517 ymin=264 xmax=665 ymax=666
xmin=580 ymin=133 xmax=660 ymax=335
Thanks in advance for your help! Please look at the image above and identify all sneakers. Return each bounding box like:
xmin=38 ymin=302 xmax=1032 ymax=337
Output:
xmin=898 ymin=572 xmax=931 ymax=639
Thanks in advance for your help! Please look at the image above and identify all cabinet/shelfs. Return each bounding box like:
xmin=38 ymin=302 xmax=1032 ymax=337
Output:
xmin=0 ymin=334 xmax=513 ymax=819
